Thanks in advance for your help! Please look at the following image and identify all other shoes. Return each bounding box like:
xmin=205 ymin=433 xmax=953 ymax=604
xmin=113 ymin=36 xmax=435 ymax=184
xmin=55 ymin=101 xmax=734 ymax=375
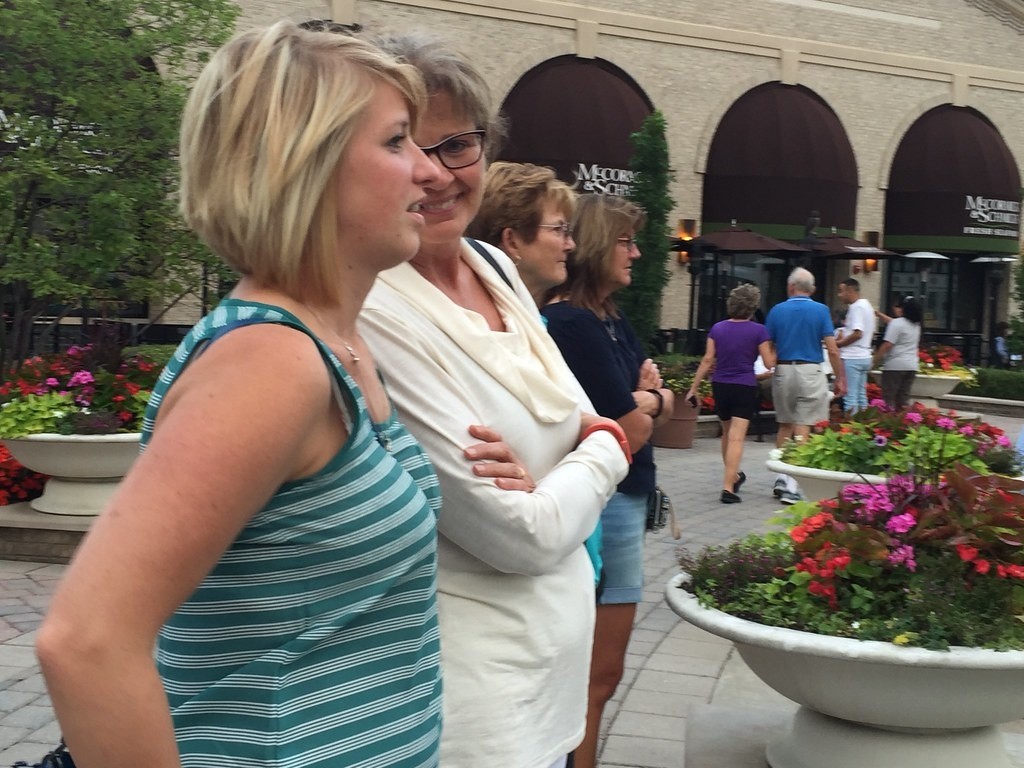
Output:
xmin=734 ymin=472 xmax=746 ymax=492
xmin=722 ymin=490 xmax=741 ymax=504
xmin=780 ymin=491 xmax=802 ymax=505
xmin=774 ymin=479 xmax=787 ymax=494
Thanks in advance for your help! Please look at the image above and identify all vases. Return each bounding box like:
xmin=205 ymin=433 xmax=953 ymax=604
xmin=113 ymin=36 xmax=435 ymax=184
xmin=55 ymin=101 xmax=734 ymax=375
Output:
xmin=871 ymin=368 xmax=961 ymax=410
xmin=649 ymin=394 xmax=696 ymax=448
xmin=3 ymin=432 xmax=141 ymax=515
xmin=664 ymin=574 xmax=1024 ymax=734
xmin=765 ymin=449 xmax=887 ymax=501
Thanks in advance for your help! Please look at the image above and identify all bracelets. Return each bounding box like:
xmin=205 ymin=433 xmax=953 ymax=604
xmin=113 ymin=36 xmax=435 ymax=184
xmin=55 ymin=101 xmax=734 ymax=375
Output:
xmin=580 ymin=423 xmax=632 ymax=463
xmin=646 ymin=389 xmax=663 ymax=418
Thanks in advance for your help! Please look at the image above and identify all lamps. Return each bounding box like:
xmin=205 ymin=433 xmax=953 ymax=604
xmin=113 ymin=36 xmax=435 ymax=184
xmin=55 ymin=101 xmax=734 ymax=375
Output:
xmin=863 ymin=229 xmax=880 ymax=274
xmin=677 ymin=218 xmax=699 ymax=264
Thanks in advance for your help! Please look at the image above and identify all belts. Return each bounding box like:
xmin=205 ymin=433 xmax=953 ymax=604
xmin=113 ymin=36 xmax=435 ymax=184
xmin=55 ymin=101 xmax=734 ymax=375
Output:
xmin=777 ymin=360 xmax=814 ymax=365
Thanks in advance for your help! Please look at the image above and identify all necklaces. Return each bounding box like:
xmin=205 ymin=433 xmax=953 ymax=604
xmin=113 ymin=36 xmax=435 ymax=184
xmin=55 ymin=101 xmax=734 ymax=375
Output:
xmin=603 ymin=316 xmax=616 ymax=341
xmin=306 ymin=302 xmax=360 ymax=361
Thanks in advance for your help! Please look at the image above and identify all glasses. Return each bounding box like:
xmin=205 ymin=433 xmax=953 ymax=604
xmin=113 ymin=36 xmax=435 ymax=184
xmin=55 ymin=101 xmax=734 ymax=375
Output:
xmin=421 ymin=128 xmax=488 ymax=169
xmin=297 ymin=19 xmax=365 ymax=37
xmin=539 ymin=222 xmax=575 ymax=239
xmin=617 ymin=235 xmax=637 ymax=251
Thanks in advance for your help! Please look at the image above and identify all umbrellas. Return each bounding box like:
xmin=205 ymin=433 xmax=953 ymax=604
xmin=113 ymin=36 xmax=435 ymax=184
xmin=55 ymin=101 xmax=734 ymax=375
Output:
xmin=668 ymin=227 xmax=812 ymax=330
xmin=778 ymin=235 xmax=905 ymax=273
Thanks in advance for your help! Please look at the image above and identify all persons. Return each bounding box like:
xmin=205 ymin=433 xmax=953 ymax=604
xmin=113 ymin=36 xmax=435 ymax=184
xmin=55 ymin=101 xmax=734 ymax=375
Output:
xmin=994 ymin=321 xmax=1011 ymax=364
xmin=685 ymin=282 xmax=778 ymax=503
xmin=33 ymin=20 xmax=442 ymax=768
xmin=541 ymin=194 xmax=674 ymax=768
xmin=356 ymin=25 xmax=629 ymax=768
xmin=836 ymin=279 xmax=876 ymax=420
xmin=462 ymin=163 xmax=603 ymax=598
xmin=765 ymin=266 xmax=846 ymax=502
xmin=754 ymin=355 xmax=775 ymax=390
xmin=835 ymin=310 xmax=847 ymax=340
xmin=874 ymin=292 xmax=922 ymax=407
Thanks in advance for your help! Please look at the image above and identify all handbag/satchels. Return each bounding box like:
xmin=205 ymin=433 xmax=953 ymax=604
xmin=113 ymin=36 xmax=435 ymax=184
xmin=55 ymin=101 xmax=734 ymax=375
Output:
xmin=647 ymin=488 xmax=670 ymax=534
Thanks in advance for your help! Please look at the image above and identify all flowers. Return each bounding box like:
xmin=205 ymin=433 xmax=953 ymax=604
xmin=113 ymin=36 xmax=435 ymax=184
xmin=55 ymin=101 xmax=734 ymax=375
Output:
xmin=651 ymin=353 xmax=717 ymax=412
xmin=876 ymin=341 xmax=979 ymax=388
xmin=783 ymin=383 xmax=1024 ymax=475
xmin=682 ymin=412 xmax=1023 ymax=652
xmin=1 ymin=343 xmax=168 ymax=430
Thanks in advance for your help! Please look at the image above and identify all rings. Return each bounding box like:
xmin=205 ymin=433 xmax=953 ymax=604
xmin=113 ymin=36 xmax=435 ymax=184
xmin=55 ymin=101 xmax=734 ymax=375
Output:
xmin=520 ymin=468 xmax=524 ymax=476
